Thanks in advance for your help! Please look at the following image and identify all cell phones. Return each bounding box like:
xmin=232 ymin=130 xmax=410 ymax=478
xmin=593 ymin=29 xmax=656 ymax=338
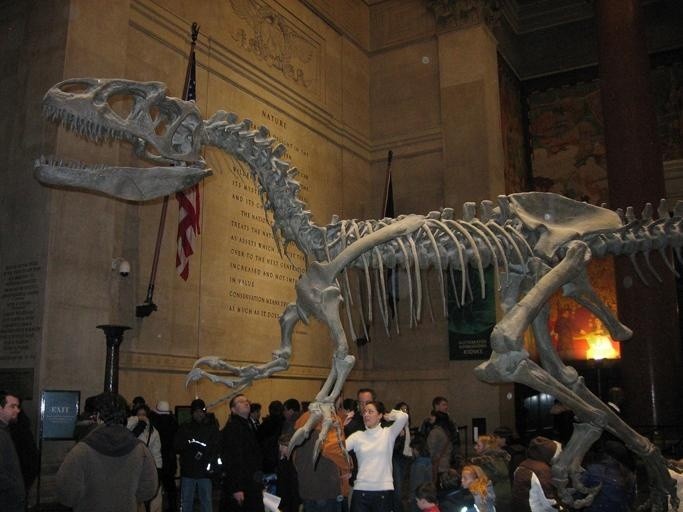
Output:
xmin=285 ymin=408 xmax=294 ymax=417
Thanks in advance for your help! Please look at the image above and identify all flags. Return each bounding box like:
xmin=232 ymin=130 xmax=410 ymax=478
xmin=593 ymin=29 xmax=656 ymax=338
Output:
xmin=173 ymin=40 xmax=199 ymax=283
xmin=383 ymin=173 xmax=399 ymax=318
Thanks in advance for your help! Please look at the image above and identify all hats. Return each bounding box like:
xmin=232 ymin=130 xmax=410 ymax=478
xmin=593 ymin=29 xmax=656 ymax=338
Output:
xmin=190 ymin=399 xmax=206 ymax=414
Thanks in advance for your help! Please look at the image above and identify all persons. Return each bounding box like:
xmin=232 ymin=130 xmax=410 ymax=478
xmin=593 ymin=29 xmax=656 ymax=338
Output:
xmin=0 ymin=383 xmax=650 ymax=511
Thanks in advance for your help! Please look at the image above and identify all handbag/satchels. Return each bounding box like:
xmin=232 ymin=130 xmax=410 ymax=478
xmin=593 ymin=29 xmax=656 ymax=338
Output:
xmin=196 ymin=444 xmax=225 ymax=479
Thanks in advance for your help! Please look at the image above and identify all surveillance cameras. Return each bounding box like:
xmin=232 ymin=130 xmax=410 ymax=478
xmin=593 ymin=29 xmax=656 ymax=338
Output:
xmin=119 ymin=261 xmax=131 ymax=277
